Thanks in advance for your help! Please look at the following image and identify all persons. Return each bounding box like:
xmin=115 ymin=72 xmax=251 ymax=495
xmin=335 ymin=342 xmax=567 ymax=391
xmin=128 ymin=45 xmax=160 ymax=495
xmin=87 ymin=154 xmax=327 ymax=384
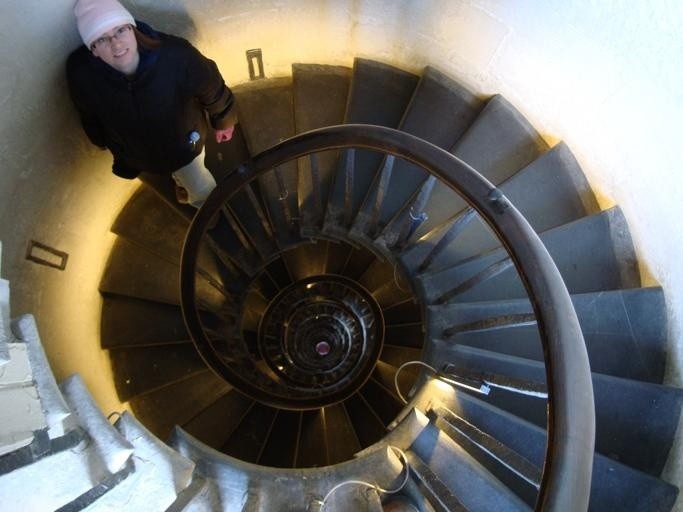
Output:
xmin=65 ymin=0 xmax=241 ymax=232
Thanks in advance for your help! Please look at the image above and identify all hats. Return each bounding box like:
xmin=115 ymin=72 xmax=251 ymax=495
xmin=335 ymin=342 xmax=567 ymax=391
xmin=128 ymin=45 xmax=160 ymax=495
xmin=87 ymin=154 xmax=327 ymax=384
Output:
xmin=74 ymin=0 xmax=137 ymax=51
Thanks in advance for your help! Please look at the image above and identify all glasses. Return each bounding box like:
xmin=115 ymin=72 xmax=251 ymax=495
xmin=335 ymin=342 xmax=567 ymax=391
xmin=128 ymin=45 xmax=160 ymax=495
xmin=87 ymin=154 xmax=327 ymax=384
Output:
xmin=94 ymin=25 xmax=131 ymax=47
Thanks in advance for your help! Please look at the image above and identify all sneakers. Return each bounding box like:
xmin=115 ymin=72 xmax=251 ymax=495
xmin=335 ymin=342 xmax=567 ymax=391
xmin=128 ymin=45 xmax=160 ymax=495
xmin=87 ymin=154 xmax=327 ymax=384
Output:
xmin=176 ymin=186 xmax=189 ymax=205
